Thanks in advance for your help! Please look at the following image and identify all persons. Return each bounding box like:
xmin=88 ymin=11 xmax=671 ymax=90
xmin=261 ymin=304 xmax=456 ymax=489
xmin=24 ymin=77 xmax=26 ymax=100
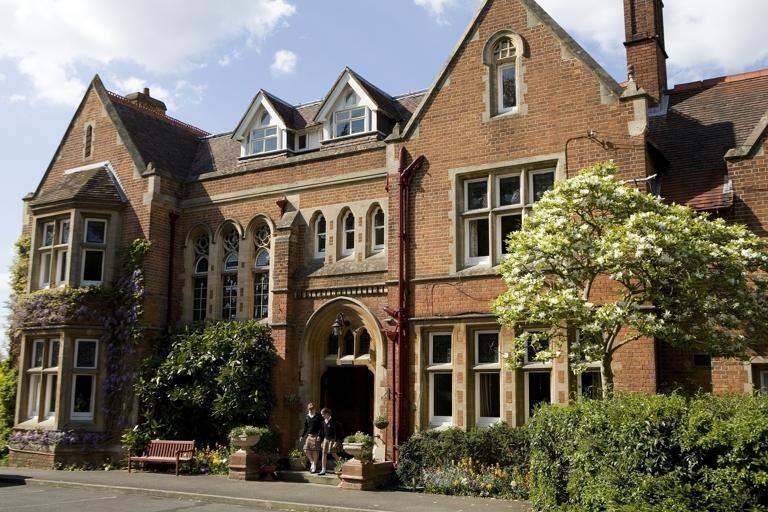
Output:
xmin=317 ymin=408 xmax=340 ymax=475
xmin=299 ymin=403 xmax=322 ymax=473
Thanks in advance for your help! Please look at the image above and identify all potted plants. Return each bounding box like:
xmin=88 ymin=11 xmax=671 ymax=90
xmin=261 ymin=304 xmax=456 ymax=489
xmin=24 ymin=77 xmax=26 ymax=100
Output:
xmin=342 ymin=429 xmax=376 ymax=460
xmin=229 ymin=424 xmax=270 ymax=451
xmin=374 ymin=415 xmax=390 ymax=429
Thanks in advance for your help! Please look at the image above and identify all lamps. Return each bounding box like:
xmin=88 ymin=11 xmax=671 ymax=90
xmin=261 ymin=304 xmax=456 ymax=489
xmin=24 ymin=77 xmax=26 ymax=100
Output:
xmin=330 ymin=311 xmax=351 ymax=337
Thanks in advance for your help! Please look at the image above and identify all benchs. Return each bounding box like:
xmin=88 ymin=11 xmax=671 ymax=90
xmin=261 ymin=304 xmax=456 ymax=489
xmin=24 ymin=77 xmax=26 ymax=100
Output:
xmin=127 ymin=438 xmax=197 ymax=476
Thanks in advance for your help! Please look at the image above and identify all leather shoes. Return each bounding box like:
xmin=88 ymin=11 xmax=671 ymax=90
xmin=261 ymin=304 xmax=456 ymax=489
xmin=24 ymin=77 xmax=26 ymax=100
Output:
xmin=310 ymin=466 xmax=325 ymax=475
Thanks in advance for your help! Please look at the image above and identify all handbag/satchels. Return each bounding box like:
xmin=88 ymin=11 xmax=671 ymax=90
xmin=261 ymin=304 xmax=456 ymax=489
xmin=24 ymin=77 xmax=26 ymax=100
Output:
xmin=306 ymin=435 xmax=316 ymax=449
